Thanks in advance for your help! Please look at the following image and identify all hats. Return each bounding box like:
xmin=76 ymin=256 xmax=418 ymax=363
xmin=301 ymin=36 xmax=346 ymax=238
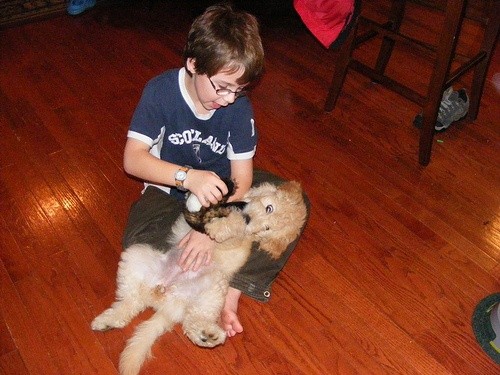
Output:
xmin=472 ymin=292 xmax=500 ymax=364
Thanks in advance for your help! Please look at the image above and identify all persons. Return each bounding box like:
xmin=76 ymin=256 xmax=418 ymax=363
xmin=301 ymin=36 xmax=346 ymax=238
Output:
xmin=122 ymin=7 xmax=310 ymax=339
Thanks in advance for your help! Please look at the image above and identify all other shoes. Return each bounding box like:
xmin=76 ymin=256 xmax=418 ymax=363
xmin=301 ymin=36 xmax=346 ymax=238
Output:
xmin=68 ymin=0 xmax=97 ymax=15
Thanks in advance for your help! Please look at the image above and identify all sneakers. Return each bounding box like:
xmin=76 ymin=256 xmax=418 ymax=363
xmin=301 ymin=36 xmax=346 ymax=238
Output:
xmin=414 ymin=85 xmax=470 ymax=131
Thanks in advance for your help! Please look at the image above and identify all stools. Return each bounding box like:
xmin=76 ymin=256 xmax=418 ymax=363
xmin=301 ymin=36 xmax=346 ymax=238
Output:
xmin=325 ymin=0 xmax=500 ymax=166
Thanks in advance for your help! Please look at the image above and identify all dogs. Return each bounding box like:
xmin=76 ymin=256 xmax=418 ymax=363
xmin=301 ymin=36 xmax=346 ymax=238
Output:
xmin=91 ymin=181 xmax=306 ymax=375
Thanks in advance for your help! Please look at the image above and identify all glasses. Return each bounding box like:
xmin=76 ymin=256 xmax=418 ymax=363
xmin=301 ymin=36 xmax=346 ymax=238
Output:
xmin=206 ymin=73 xmax=248 ymax=97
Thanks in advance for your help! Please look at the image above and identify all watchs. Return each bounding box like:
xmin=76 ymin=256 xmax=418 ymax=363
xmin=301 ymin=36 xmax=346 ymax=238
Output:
xmin=174 ymin=164 xmax=193 ymax=191
